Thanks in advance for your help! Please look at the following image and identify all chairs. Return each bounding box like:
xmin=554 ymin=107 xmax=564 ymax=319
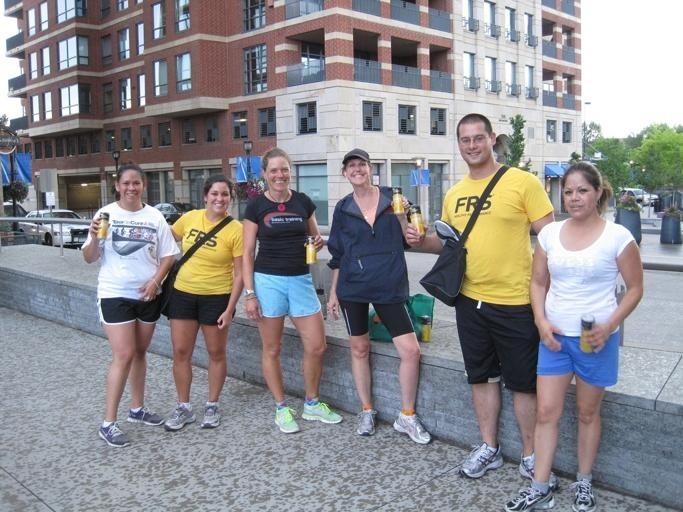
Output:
xmin=518 ymin=452 xmax=561 ymax=491
xmin=200 ymin=405 xmax=222 ymax=428
xmin=303 ymin=401 xmax=343 ymax=424
xmin=274 ymin=407 xmax=301 ymax=434
xmin=357 ymin=408 xmax=378 ymax=436
xmin=126 ymin=407 xmax=165 ymax=427
xmin=393 ymin=412 xmax=432 ymax=445
xmin=98 ymin=421 xmax=131 ymax=448
xmin=504 ymin=482 xmax=555 ymax=512
xmin=459 ymin=439 xmax=504 ymax=479
xmin=572 ymin=476 xmax=597 ymax=512
xmin=165 ymin=404 xmax=196 ymax=431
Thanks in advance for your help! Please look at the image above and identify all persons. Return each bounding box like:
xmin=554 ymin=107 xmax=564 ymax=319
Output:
xmin=326 ymin=147 xmax=432 ymax=446
xmin=80 ymin=162 xmax=164 ymax=448
xmin=159 ymin=173 xmax=244 ymax=432
xmin=405 ymin=113 xmax=560 ymax=492
xmin=505 ymin=160 xmax=644 ymax=512
xmin=242 ymin=147 xmax=343 ymax=434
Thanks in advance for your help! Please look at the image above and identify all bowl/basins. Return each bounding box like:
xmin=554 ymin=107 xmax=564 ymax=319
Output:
xmin=202 ymin=213 xmax=207 ymax=237
xmin=268 ymin=190 xmax=291 ymax=212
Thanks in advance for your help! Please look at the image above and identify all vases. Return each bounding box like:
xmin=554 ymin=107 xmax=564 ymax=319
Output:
xmin=616 ymin=195 xmax=641 ymax=213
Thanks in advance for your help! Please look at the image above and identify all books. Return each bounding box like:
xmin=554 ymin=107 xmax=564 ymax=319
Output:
xmin=367 ymin=294 xmax=434 ymax=342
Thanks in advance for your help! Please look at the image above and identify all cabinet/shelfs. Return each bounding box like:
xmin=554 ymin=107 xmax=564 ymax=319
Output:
xmin=315 ymin=289 xmax=328 ymax=320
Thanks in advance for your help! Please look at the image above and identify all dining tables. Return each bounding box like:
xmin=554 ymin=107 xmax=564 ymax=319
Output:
xmin=659 ymin=206 xmax=681 ymax=244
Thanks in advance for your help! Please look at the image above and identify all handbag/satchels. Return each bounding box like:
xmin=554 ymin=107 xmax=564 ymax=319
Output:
xmin=245 ymin=290 xmax=255 ymax=297
xmin=152 ymin=278 xmax=159 ymax=290
xmin=246 ymin=296 xmax=256 ymax=300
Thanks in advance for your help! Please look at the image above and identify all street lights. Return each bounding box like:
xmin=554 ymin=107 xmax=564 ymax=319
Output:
xmin=158 ymin=256 xmax=180 ymax=314
xmin=365 ymin=292 xmax=435 ymax=343
xmin=418 ymin=238 xmax=468 ymax=307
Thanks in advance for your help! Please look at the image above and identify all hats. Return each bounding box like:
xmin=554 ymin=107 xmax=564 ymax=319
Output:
xmin=16 ymin=207 xmax=93 ymax=249
xmin=150 ymin=202 xmax=201 ymax=226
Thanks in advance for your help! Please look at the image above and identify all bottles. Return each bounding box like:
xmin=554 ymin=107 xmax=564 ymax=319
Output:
xmin=342 ymin=149 xmax=369 ymax=164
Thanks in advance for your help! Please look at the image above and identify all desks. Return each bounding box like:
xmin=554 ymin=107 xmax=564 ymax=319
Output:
xmin=243 ymin=139 xmax=256 ymax=184
xmin=583 ymin=98 xmax=593 ymax=156
xmin=34 ymin=171 xmax=40 ymax=232
xmin=629 ymin=160 xmax=647 ymax=214
xmin=111 ymin=148 xmax=122 ymax=200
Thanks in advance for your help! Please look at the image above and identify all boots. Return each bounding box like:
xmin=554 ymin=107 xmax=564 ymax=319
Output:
xmin=617 ymin=188 xmax=659 ymax=208
xmin=3 ymin=199 xmax=28 ymax=234
